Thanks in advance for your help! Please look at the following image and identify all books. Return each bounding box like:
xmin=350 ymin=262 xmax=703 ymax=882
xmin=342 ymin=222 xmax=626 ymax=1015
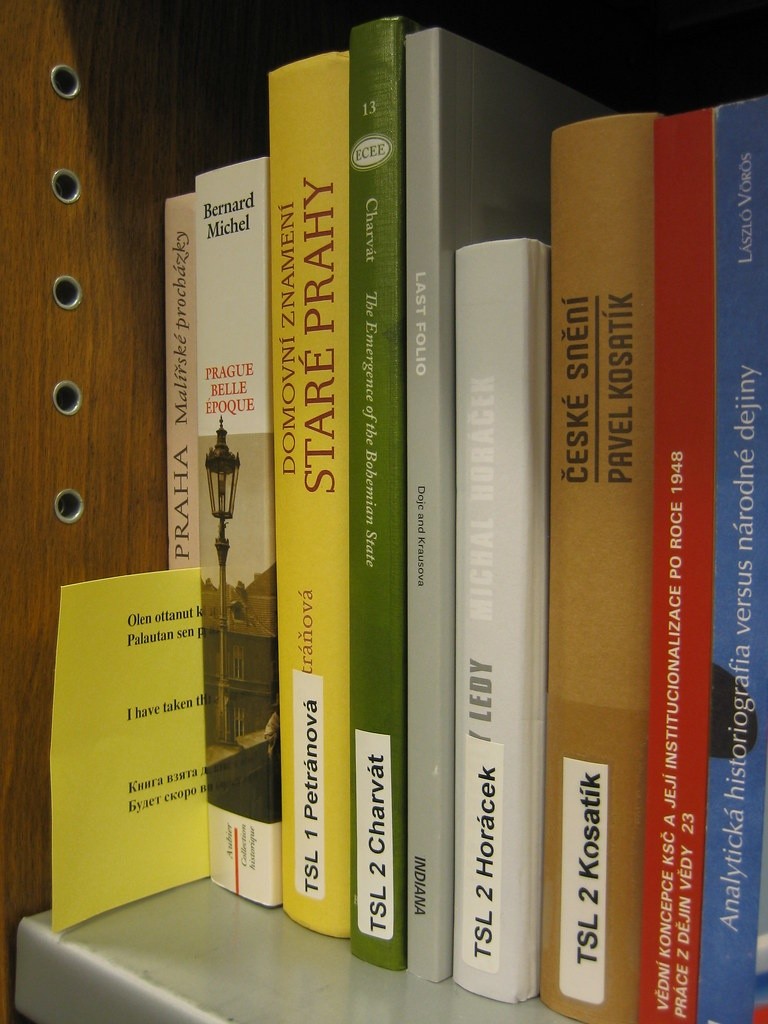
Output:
xmin=165 ymin=14 xmax=768 ymax=1024
